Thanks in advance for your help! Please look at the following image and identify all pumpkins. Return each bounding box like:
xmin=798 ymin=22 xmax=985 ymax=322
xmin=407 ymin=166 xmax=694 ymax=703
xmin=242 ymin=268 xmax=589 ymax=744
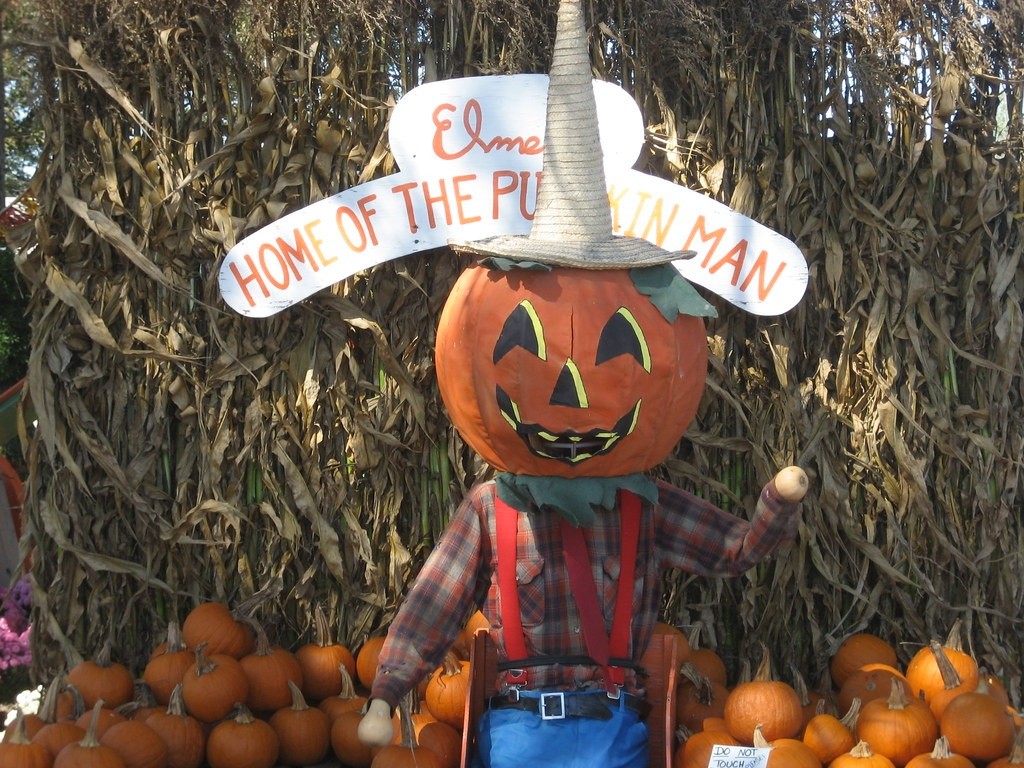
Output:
xmin=640 ymin=622 xmax=1024 ymax=768
xmin=0 ymin=599 xmax=491 ymax=768
xmin=434 ymin=256 xmax=708 ymax=477
